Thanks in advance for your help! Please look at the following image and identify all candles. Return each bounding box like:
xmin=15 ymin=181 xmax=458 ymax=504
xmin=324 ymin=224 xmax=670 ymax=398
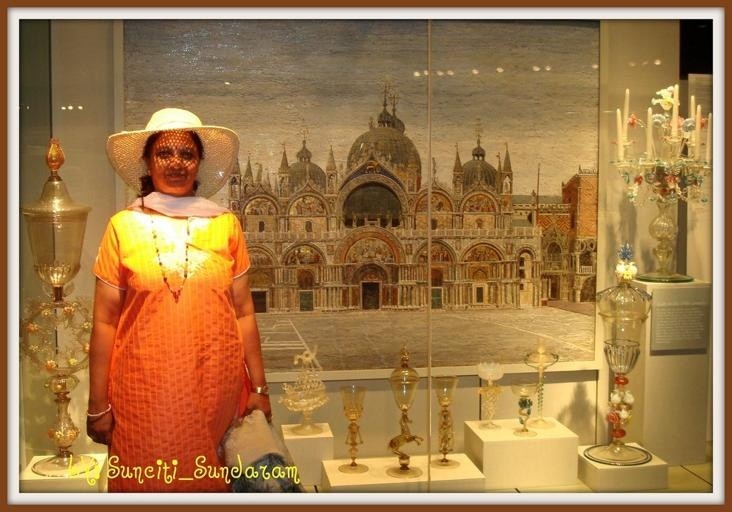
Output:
xmin=616 ymin=85 xmax=712 ymax=165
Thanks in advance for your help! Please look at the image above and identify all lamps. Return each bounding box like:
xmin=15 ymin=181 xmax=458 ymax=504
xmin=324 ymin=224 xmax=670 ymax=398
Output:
xmin=278 ymin=346 xmax=558 ymax=479
xmin=583 ymin=244 xmax=653 ymax=467
xmin=21 ymin=141 xmax=99 ymax=478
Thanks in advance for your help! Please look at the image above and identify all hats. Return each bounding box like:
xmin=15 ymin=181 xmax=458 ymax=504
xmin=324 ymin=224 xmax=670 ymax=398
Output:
xmin=106 ymin=107 xmax=239 ymax=199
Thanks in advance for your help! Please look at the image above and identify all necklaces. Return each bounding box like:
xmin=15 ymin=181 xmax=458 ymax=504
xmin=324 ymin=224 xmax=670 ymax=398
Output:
xmin=149 ymin=212 xmax=191 ymax=300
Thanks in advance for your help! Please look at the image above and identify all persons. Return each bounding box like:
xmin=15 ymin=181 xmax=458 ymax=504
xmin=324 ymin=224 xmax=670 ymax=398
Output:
xmin=85 ymin=106 xmax=273 ymax=494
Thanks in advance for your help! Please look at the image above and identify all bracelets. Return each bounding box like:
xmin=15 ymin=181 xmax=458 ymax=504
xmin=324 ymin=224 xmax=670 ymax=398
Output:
xmin=251 ymin=385 xmax=269 ymax=395
xmin=86 ymin=404 xmax=112 ymax=417
